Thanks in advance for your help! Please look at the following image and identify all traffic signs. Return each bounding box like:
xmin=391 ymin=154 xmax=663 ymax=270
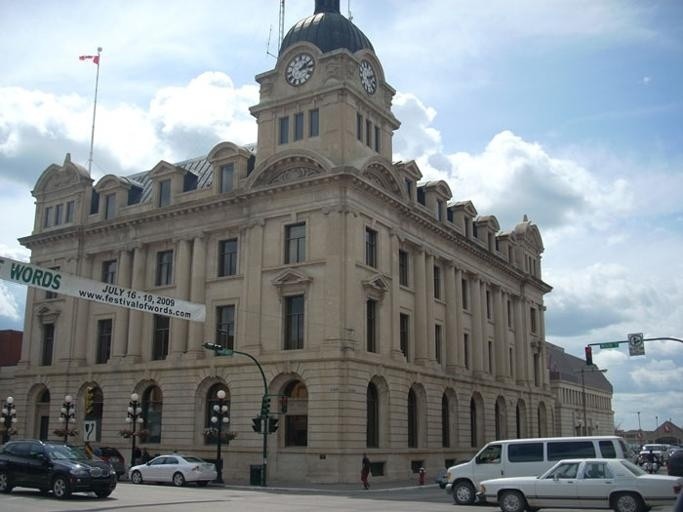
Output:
xmin=600 ymin=342 xmax=619 ymax=349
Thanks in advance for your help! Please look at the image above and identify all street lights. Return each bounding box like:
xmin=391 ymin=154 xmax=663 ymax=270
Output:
xmin=203 ymin=339 xmax=280 ymax=486
xmin=0 ymin=395 xmax=18 ymax=445
xmin=57 ymin=393 xmax=78 ymax=445
xmin=209 ymin=389 xmax=230 ymax=484
xmin=123 ymin=391 xmax=146 ymax=467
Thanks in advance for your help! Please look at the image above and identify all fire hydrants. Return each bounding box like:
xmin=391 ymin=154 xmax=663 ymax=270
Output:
xmin=416 ymin=466 xmax=427 ymax=487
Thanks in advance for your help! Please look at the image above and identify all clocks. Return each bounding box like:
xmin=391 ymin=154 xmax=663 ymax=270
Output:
xmin=358 ymin=58 xmax=377 ymax=95
xmin=283 ymin=53 xmax=315 ymax=87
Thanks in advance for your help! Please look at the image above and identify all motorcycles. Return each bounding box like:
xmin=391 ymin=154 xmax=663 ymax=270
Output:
xmin=640 ymin=456 xmax=658 ymax=474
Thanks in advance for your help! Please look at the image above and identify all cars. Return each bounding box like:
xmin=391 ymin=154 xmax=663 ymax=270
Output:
xmin=476 ymin=456 xmax=682 ymax=512
xmin=128 ymin=455 xmax=220 ymax=486
xmin=669 ymin=445 xmax=683 ymax=456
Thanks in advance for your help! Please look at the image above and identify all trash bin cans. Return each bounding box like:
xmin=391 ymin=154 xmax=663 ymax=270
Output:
xmin=250 ymin=464 xmax=266 ymax=486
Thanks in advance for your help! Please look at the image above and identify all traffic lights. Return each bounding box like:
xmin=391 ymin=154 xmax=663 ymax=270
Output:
xmin=263 ymin=397 xmax=272 ymax=419
xmin=584 ymin=347 xmax=591 ymax=365
xmin=270 ymin=418 xmax=279 ymax=435
xmin=251 ymin=417 xmax=260 ymax=431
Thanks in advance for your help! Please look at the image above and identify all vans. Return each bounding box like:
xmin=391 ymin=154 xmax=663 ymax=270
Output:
xmin=437 ymin=435 xmax=633 ymax=504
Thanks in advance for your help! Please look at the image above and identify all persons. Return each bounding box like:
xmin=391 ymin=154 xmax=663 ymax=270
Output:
xmin=646 ymin=449 xmax=657 ymax=463
xmin=360 ymin=453 xmax=371 ymax=489
xmin=135 ymin=445 xmax=141 ymax=465
xmin=638 ymin=454 xmax=644 ymax=466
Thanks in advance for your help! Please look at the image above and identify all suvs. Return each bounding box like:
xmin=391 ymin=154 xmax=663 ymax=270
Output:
xmin=71 ymin=445 xmax=126 ymax=480
xmin=1 ymin=440 xmax=116 ymax=501
xmin=640 ymin=443 xmax=670 ymax=467
